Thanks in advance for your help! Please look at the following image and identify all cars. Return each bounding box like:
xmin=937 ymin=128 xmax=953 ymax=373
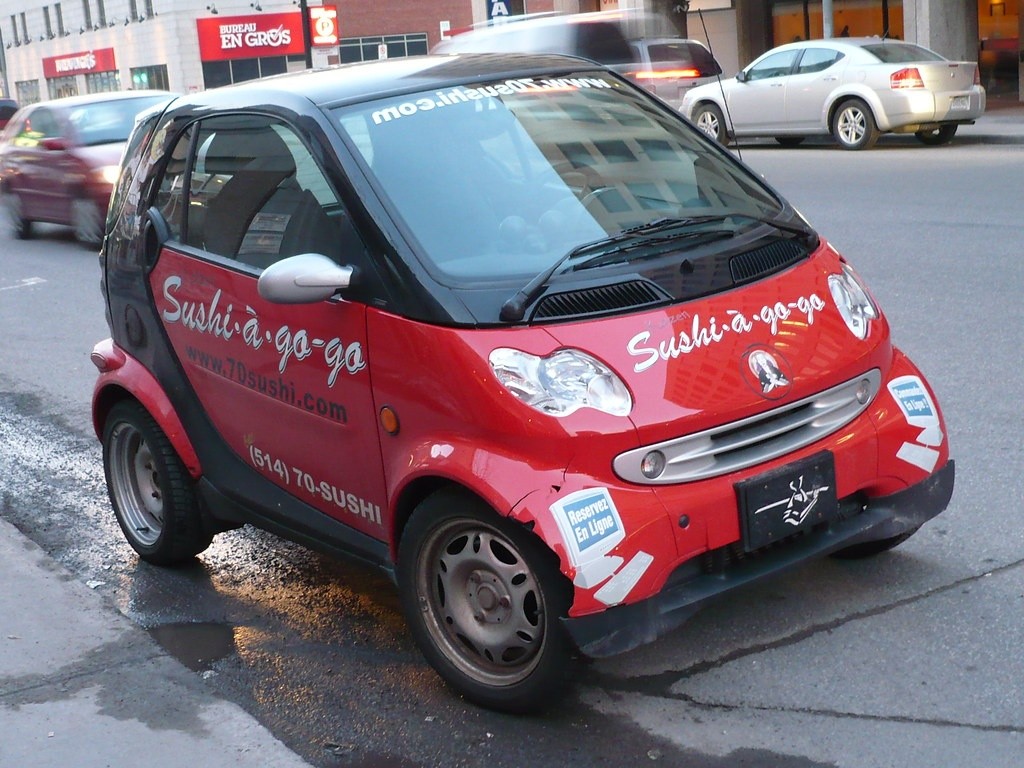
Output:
xmin=0 ymin=89 xmax=187 ymax=249
xmin=1 ymin=98 xmax=21 ymax=132
xmin=676 ymin=36 xmax=987 ymax=150
xmin=632 ymin=39 xmax=724 ymax=98
xmin=91 ymin=52 xmax=957 ymax=717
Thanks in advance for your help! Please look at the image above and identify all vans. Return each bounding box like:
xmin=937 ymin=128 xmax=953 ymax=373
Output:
xmin=426 ymin=10 xmax=700 ymax=114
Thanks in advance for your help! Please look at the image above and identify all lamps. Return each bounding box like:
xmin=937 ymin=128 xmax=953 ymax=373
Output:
xmin=207 ymin=3 xmax=218 ymax=14
xmin=7 ymin=12 xmax=157 ymax=49
xmin=250 ymin=0 xmax=262 ymax=11
xmin=293 ymin=0 xmax=301 ymax=8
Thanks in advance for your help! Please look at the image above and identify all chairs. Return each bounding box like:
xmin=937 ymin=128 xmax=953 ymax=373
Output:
xmin=194 ymin=126 xmax=340 ymax=269
